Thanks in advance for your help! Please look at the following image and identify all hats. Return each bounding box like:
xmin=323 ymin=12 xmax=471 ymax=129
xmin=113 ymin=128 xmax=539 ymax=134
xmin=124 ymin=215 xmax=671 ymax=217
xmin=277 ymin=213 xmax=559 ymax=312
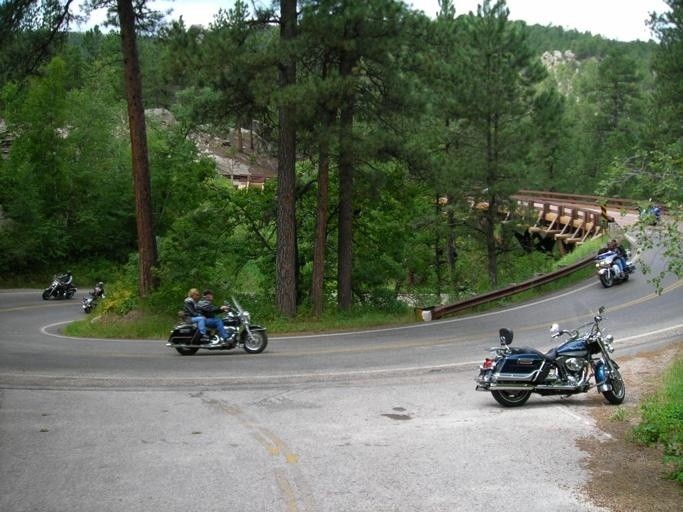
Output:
xmin=204 ymin=289 xmax=215 ymax=296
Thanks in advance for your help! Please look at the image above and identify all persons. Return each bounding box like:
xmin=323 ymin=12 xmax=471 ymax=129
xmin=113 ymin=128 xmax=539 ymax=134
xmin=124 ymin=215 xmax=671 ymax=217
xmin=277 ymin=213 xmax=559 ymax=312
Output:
xmin=95 ymin=281 xmax=105 ymax=299
xmin=607 ymin=239 xmax=627 ymax=277
xmin=184 ymin=288 xmax=210 ymax=339
xmin=57 ymin=269 xmax=73 ymax=293
xmin=197 ymin=289 xmax=233 ymax=341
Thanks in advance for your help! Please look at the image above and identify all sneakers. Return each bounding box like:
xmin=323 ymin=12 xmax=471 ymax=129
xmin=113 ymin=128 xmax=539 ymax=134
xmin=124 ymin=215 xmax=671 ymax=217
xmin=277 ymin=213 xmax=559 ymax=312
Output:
xmin=224 ymin=337 xmax=236 ymax=342
xmin=201 ymin=334 xmax=210 ymax=339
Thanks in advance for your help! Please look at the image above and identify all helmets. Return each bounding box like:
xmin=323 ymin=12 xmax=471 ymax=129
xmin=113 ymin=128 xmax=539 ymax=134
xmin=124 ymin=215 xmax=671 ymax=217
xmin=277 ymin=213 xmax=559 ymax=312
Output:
xmin=66 ymin=270 xmax=72 ymax=276
xmin=98 ymin=282 xmax=104 ymax=287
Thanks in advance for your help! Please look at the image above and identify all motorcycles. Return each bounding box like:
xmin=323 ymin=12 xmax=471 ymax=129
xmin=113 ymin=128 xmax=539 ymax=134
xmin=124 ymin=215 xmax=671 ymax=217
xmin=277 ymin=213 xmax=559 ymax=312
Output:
xmin=42 ymin=275 xmax=76 ymax=300
xmin=82 ymin=291 xmax=106 ymax=313
xmin=634 ymin=198 xmax=661 ymax=226
xmin=593 ymin=246 xmax=634 ymax=287
xmin=166 ymin=297 xmax=268 ymax=355
xmin=476 ymin=307 xmax=625 ymax=406
